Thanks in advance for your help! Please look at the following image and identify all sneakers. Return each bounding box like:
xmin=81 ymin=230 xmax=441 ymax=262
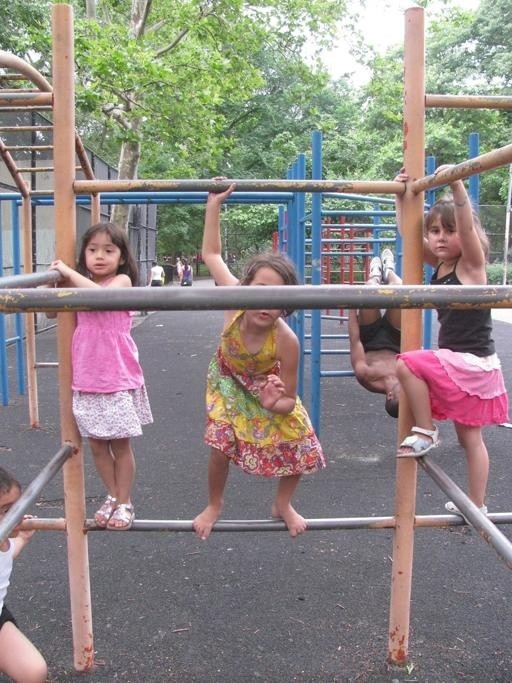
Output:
xmin=368 ymin=248 xmax=395 ymax=283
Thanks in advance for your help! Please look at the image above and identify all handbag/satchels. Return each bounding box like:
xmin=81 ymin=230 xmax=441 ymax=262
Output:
xmin=181 ymin=279 xmax=192 ymax=286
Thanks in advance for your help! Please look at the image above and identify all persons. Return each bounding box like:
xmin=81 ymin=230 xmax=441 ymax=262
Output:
xmin=192 ymin=173 xmax=326 ymax=540
xmin=392 ymin=164 xmax=510 ymax=527
xmin=172 ymin=256 xmax=184 ymax=282
xmin=179 ymin=258 xmax=192 ymax=285
xmin=346 ymin=248 xmax=403 ymax=419
xmin=44 ymin=218 xmax=155 ymax=531
xmin=0 ymin=466 xmax=49 ymax=682
xmin=145 ymin=260 xmax=166 ymax=285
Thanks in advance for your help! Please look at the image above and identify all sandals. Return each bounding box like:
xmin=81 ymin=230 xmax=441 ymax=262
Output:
xmin=444 ymin=500 xmax=489 ymax=527
xmin=93 ymin=493 xmax=135 ymax=532
xmin=396 ymin=423 xmax=443 ymax=460
